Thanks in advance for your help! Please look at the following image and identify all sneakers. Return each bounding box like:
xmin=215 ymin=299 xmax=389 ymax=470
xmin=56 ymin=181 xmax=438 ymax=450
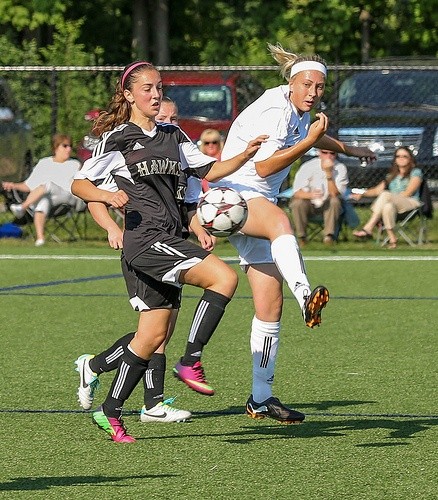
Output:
xmin=92 ymin=405 xmax=137 ymax=443
xmin=246 ymin=393 xmax=305 ymax=424
xmin=173 ymin=360 xmax=215 ymax=396
xmin=301 ymin=286 xmax=330 ymax=329
xmin=140 ymin=395 xmax=193 ymax=424
xmin=73 ymin=353 xmax=100 ymax=410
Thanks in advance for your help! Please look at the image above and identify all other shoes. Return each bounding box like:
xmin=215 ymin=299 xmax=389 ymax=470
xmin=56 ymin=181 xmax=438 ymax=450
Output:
xmin=298 ymin=238 xmax=307 ymax=248
xmin=382 ymin=242 xmax=397 ymax=249
xmin=10 ymin=204 xmax=25 ymax=220
xmin=34 ymin=239 xmax=45 ymax=247
xmin=351 ymin=229 xmax=374 ymax=240
xmin=323 ymin=235 xmax=334 ymax=243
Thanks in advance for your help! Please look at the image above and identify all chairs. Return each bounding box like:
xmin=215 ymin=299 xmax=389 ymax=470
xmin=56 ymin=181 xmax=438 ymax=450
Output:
xmin=277 ymin=187 xmax=351 ymax=243
xmin=3 ymin=188 xmax=86 ymax=245
xmin=375 ymin=173 xmax=434 ymax=246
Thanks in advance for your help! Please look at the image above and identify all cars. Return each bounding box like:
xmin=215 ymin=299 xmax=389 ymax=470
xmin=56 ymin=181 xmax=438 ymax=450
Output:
xmin=75 ymin=72 xmax=265 ymax=165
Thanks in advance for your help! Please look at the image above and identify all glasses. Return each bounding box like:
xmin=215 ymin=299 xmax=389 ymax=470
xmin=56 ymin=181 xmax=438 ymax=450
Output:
xmin=321 ymin=150 xmax=336 ymax=156
xmin=202 ymin=140 xmax=221 ymax=146
xmin=60 ymin=143 xmax=72 ymax=148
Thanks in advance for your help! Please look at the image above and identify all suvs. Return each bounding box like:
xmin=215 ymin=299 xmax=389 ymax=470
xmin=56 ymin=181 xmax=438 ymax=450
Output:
xmin=300 ymin=56 xmax=438 ymax=199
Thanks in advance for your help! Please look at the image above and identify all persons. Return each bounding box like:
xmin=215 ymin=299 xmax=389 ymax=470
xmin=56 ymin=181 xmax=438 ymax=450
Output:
xmin=76 ymin=96 xmax=212 ymax=425
xmin=212 ymin=45 xmax=379 ymax=423
xmin=198 ymin=128 xmax=224 ymax=193
xmin=71 ymin=60 xmax=270 ymax=443
xmin=351 ymin=146 xmax=425 ymax=250
xmin=2 ymin=135 xmax=86 ymax=248
xmin=290 ymin=147 xmax=351 ymax=246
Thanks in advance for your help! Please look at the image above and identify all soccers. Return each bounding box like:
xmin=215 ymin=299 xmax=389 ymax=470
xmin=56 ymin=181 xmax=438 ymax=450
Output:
xmin=197 ymin=186 xmax=249 ymax=237
